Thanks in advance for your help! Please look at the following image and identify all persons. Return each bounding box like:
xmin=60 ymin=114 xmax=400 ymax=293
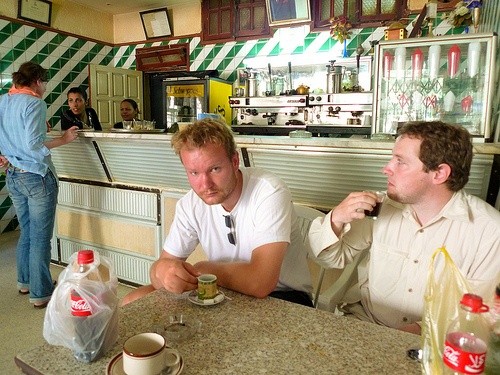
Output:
xmin=113 ymin=99 xmax=141 ymax=128
xmin=61 ymin=87 xmax=102 ymax=131
xmin=0 ymin=62 xmax=79 ymax=308
xmin=305 ymin=120 xmax=500 ymax=338
xmin=121 ymin=118 xmax=315 ymax=308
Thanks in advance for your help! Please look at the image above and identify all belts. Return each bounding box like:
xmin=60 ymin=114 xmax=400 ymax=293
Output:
xmin=8 ymin=165 xmax=28 ymax=173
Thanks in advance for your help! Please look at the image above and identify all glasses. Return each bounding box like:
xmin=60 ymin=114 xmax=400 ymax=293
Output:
xmin=223 ymin=214 xmax=237 ymax=246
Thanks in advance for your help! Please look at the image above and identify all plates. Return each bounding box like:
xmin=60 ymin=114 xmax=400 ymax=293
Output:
xmin=189 ymin=288 xmax=225 ymax=306
xmin=106 ymin=345 xmax=185 ymax=375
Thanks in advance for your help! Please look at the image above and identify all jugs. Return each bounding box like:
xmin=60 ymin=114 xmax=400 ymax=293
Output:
xmin=325 ymin=65 xmax=346 ymax=93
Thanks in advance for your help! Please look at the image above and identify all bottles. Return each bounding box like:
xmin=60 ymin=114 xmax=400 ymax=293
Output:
xmin=68 ymin=249 xmax=105 ymax=363
xmin=443 ymin=294 xmax=489 ymax=375
xmin=485 ymin=284 xmax=500 ymax=375
xmin=239 ymin=67 xmax=258 ymax=98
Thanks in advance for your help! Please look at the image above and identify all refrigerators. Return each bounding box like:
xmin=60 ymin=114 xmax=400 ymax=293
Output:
xmin=161 ymin=77 xmax=234 ymax=133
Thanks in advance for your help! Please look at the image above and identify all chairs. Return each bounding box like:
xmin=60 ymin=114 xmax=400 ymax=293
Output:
xmin=295 ymin=204 xmax=328 ymax=310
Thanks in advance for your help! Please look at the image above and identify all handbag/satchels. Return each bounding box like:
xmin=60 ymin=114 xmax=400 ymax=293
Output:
xmin=43 ymin=251 xmax=121 ymax=362
xmin=420 ymin=246 xmax=500 ymax=375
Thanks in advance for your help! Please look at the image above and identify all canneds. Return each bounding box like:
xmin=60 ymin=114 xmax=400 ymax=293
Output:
xmin=184 ymin=108 xmax=194 ymax=115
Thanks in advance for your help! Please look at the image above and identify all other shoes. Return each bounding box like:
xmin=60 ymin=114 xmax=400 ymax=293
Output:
xmin=18 ymin=280 xmax=57 ymax=308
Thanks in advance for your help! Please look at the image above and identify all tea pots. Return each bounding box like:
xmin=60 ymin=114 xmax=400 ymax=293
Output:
xmin=295 ymin=83 xmax=310 ymax=95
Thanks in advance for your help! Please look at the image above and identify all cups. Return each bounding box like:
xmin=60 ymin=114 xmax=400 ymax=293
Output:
xmin=123 ymin=332 xmax=182 ymax=375
xmin=362 ymin=190 xmax=386 ymax=221
xmin=123 ymin=120 xmax=156 ymax=131
xmin=193 ymin=274 xmax=217 ymax=300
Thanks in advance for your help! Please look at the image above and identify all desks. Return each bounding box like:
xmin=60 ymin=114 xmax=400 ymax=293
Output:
xmin=14 ymin=284 xmax=500 ymax=375
